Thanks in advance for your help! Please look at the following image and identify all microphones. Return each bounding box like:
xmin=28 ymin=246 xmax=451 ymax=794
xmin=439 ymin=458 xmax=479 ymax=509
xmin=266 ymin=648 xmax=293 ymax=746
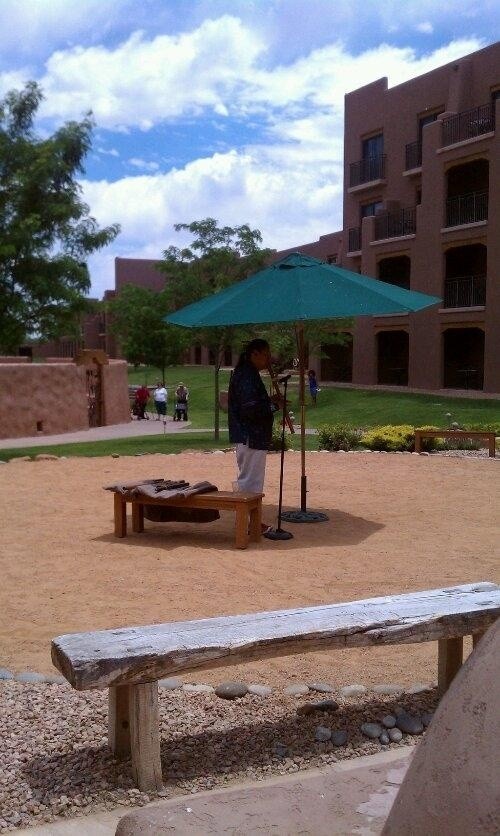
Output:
xmin=280 ymin=375 xmax=291 ymax=382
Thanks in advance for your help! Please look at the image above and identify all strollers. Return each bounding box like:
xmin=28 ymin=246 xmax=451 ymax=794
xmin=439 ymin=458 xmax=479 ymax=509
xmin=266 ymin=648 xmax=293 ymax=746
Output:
xmin=173 ymin=396 xmax=189 ymax=422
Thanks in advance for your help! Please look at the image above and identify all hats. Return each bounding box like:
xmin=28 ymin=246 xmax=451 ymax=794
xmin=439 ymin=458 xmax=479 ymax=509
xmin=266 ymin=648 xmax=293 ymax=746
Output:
xmin=178 ymin=382 xmax=184 ymax=387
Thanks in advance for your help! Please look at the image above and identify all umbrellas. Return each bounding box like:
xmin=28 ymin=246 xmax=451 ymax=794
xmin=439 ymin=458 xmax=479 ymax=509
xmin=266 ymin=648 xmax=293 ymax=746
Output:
xmin=160 ymin=250 xmax=445 ymax=514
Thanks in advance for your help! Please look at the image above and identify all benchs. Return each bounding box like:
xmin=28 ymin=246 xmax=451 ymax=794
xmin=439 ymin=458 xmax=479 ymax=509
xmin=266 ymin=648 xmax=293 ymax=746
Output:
xmin=409 ymin=425 xmax=497 ymax=457
xmin=107 ymin=486 xmax=268 ymax=552
xmin=47 ymin=574 xmax=500 ymax=793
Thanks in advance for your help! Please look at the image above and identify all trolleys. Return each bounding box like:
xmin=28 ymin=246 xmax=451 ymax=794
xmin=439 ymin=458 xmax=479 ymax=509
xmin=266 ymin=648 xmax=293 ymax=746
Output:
xmin=130 ymin=399 xmax=149 ymax=421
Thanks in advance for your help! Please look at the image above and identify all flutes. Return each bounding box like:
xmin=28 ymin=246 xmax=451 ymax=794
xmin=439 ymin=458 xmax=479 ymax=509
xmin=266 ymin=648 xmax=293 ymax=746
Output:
xmin=267 ymin=364 xmax=295 ymax=434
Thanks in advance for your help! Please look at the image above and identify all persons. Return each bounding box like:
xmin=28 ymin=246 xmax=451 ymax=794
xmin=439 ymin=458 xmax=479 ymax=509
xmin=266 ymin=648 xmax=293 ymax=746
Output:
xmin=227 ymin=338 xmax=293 ymax=538
xmin=308 ymin=368 xmax=320 ymax=404
xmin=134 ymin=379 xmax=192 ymax=423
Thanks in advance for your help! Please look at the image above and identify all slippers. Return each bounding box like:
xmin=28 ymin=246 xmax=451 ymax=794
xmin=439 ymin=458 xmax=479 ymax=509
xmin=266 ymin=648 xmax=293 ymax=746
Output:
xmin=248 ymin=526 xmax=274 ymax=534
